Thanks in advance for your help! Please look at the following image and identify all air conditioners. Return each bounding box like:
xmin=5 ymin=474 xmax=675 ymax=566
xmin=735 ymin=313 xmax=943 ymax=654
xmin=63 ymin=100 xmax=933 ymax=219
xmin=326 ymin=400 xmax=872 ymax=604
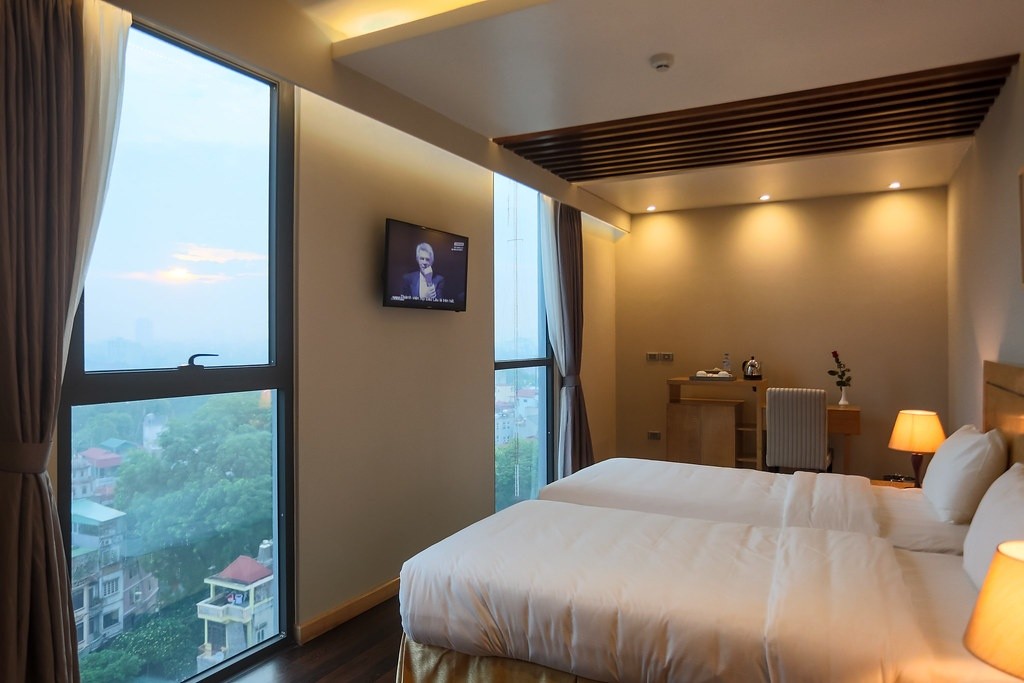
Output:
xmin=102 ymin=539 xmax=111 ymax=546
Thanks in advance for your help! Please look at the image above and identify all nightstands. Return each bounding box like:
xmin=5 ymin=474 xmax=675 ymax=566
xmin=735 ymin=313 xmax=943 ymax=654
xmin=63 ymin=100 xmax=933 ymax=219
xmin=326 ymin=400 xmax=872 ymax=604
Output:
xmin=870 ymin=480 xmax=914 ymax=489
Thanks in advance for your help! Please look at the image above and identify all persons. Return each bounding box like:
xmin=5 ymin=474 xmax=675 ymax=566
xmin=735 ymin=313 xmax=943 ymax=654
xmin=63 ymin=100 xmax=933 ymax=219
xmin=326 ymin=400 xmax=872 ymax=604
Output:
xmin=398 ymin=242 xmax=445 ymax=300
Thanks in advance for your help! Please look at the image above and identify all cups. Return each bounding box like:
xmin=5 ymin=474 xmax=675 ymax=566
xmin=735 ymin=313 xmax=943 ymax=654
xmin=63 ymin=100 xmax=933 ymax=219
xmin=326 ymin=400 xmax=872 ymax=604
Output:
xmin=718 ymin=371 xmax=732 ymax=378
xmin=696 ymin=371 xmax=707 ymax=377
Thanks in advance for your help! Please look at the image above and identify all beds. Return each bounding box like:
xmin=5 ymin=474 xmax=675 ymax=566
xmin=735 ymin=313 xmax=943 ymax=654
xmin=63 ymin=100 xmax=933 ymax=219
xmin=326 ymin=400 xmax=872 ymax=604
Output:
xmin=536 ymin=359 xmax=1024 ymax=555
xmin=395 ymin=500 xmax=1022 ymax=683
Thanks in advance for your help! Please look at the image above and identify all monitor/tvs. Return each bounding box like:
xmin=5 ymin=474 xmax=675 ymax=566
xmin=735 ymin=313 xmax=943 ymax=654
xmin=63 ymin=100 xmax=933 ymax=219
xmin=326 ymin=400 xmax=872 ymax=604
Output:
xmin=383 ymin=218 xmax=469 ymax=312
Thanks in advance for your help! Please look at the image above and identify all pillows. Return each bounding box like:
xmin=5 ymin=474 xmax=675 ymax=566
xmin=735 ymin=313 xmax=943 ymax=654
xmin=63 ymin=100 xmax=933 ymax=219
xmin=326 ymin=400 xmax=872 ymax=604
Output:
xmin=962 ymin=462 xmax=1024 ymax=591
xmin=922 ymin=424 xmax=1008 ymax=525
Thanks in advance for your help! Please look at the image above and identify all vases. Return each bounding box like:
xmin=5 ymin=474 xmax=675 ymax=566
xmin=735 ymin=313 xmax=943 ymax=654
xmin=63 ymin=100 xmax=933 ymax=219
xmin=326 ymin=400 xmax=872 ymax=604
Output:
xmin=838 ymin=386 xmax=849 ymax=408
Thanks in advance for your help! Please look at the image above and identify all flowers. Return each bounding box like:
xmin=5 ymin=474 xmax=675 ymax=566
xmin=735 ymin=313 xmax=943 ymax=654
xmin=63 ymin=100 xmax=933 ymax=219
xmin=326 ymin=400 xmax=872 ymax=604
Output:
xmin=827 ymin=351 xmax=852 ymax=392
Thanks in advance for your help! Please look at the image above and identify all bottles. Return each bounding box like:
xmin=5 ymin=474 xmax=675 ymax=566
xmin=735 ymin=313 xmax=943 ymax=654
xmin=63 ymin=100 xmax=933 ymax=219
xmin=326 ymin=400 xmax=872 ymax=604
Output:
xmin=722 ymin=353 xmax=731 ymax=371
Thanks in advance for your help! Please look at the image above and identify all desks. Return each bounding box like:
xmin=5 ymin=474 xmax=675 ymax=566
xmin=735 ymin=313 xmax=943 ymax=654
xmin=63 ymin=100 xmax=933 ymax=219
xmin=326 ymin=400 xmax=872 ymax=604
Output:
xmin=761 ymin=404 xmax=860 ymax=475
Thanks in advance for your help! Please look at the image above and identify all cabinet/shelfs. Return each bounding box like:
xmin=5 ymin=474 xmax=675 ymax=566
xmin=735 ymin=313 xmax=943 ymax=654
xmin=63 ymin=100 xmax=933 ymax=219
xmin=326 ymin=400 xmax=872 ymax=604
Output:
xmin=666 ymin=377 xmax=769 ymax=471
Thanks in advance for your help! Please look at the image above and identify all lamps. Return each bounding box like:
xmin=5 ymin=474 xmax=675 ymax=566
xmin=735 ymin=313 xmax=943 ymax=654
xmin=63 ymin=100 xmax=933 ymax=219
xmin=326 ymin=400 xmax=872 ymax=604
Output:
xmin=888 ymin=410 xmax=946 ymax=489
xmin=963 ymin=541 xmax=1024 ymax=680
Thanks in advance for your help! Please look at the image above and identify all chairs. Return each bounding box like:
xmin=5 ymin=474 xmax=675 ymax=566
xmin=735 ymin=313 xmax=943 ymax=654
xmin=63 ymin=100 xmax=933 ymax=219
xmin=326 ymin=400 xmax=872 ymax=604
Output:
xmin=224 ymin=591 xmax=248 ymax=604
xmin=766 ymin=387 xmax=834 ymax=473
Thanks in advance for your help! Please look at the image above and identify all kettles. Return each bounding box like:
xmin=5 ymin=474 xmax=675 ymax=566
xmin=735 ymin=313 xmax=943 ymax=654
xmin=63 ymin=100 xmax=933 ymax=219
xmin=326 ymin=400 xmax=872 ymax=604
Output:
xmin=741 ymin=356 xmax=763 ymax=380
